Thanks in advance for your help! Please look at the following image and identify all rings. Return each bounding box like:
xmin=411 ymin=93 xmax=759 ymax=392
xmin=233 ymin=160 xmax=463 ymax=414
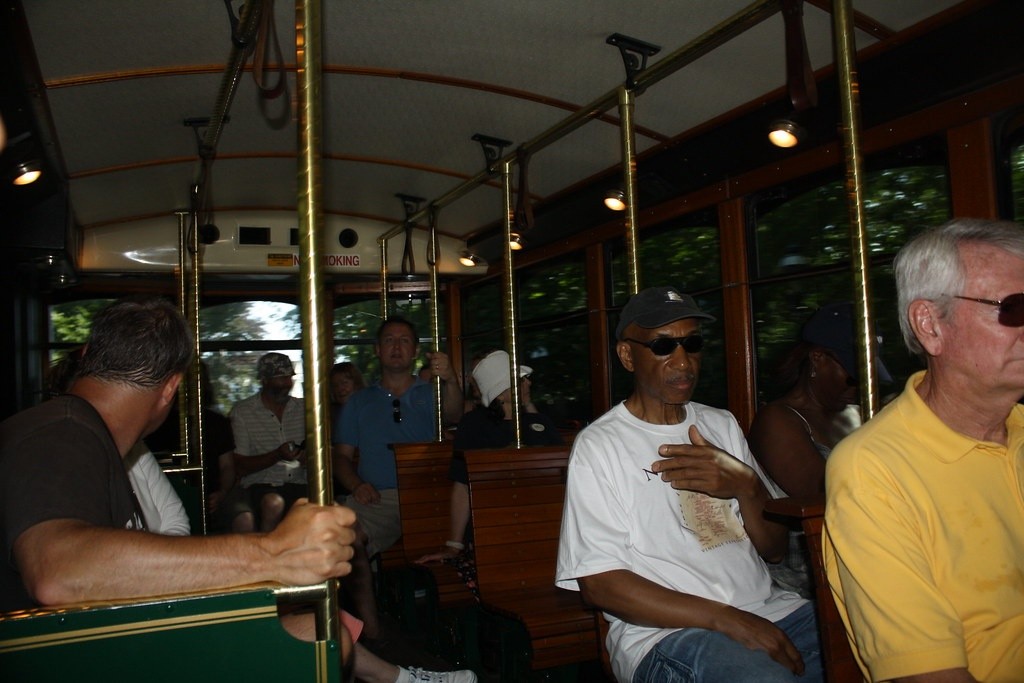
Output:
xmin=436 ymin=365 xmax=439 ymax=369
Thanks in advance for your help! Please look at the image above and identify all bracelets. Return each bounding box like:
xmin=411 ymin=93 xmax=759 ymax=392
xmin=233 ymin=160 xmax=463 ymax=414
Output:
xmin=445 ymin=541 xmax=465 ymax=550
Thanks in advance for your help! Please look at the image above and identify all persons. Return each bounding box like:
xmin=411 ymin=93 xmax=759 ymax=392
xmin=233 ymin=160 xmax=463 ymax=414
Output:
xmin=0 ymin=295 xmax=568 ymax=683
xmin=748 ymin=301 xmax=863 ymax=595
xmin=554 ymin=287 xmax=825 ymax=683
xmin=821 ymin=218 xmax=1024 ymax=683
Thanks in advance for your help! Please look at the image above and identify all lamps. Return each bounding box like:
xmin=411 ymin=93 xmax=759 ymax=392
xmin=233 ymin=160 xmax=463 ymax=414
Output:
xmin=604 ymin=182 xmax=627 ymax=211
xmin=460 ymin=250 xmax=481 ymax=266
xmin=767 ymin=97 xmax=799 ymax=148
xmin=12 ymin=158 xmax=43 ymax=185
xmin=510 ymin=231 xmax=528 ymax=250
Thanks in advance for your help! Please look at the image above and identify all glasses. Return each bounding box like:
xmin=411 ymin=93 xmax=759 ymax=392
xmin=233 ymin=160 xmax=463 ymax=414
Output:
xmin=825 ymin=350 xmax=859 ymax=386
xmin=392 ymin=398 xmax=402 ymax=425
xmin=624 ymin=334 xmax=705 ymax=355
xmin=940 ymin=291 xmax=1024 ymax=327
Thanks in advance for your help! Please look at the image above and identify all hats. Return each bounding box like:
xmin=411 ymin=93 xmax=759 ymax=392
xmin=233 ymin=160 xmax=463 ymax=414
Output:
xmin=805 ymin=303 xmax=899 ymax=390
xmin=618 ymin=285 xmax=717 ymax=331
xmin=258 ymin=352 xmax=296 ymax=379
xmin=472 ymin=351 xmax=534 ymax=407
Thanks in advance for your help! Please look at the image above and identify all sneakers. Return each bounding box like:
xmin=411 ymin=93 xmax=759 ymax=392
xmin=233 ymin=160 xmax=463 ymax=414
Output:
xmin=408 ymin=666 xmax=478 ymax=683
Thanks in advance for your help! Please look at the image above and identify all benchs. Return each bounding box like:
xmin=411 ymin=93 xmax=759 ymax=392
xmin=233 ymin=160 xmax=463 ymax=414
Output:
xmin=378 ymin=440 xmax=869 ymax=683
